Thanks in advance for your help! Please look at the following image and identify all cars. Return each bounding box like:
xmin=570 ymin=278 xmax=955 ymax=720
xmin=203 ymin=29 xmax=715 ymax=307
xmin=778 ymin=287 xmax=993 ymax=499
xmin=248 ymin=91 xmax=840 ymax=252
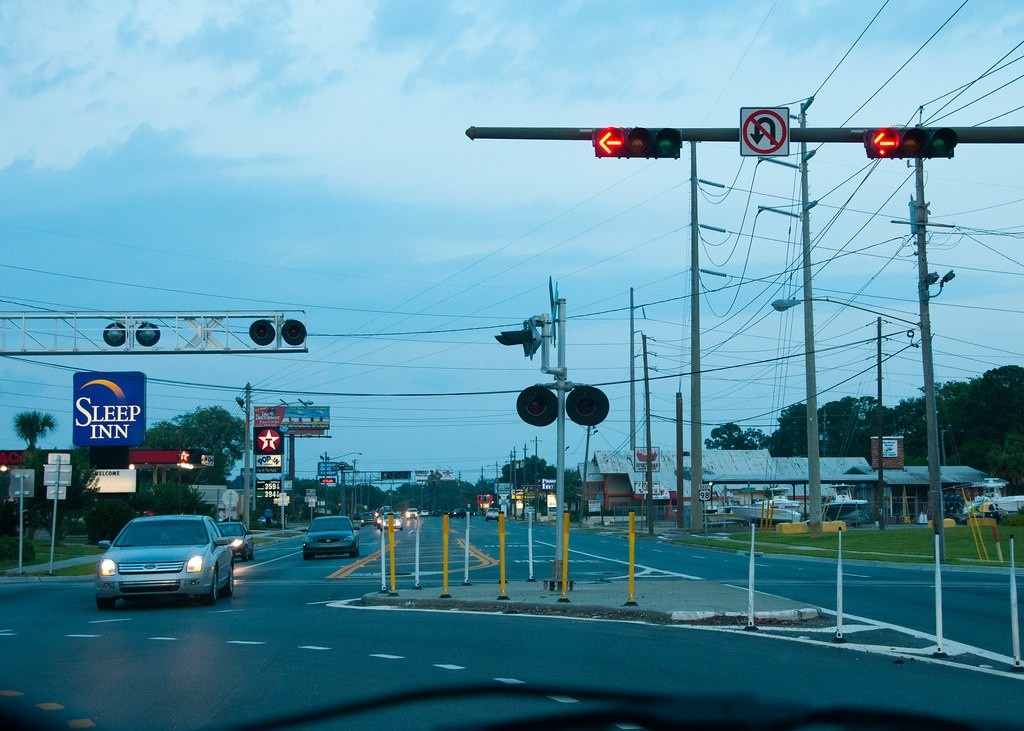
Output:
xmin=301 ymin=516 xmax=361 ymax=560
xmin=216 ymin=521 xmax=254 ymax=561
xmin=95 ymin=514 xmax=246 ymax=610
xmin=354 ymin=505 xmax=476 ymax=532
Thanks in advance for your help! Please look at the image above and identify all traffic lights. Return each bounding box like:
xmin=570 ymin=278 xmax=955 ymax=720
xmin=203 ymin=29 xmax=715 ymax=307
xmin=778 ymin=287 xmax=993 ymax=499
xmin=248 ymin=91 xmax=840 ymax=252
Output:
xmin=863 ymin=127 xmax=958 ymax=160
xmin=494 ymin=320 xmax=536 ymax=357
xmin=566 ymin=385 xmax=610 ymax=426
xmin=249 ymin=320 xmax=276 ymax=346
xmin=501 ymin=318 xmax=543 ymax=361
xmin=591 ymin=126 xmax=684 ymax=160
xmin=280 ymin=318 xmax=307 ymax=346
xmin=516 ymin=386 xmax=558 ymax=427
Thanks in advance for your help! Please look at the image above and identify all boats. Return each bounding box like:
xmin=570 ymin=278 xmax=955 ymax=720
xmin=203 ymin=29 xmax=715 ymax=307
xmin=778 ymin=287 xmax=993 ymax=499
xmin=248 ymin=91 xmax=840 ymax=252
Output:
xmin=702 ymin=482 xmax=870 ymax=528
xmin=941 ymin=477 xmax=1024 ymax=524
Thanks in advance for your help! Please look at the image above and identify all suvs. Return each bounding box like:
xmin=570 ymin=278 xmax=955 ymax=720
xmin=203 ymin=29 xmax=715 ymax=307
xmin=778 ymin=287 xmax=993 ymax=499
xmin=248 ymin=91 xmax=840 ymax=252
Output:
xmin=484 ymin=508 xmax=501 ymax=522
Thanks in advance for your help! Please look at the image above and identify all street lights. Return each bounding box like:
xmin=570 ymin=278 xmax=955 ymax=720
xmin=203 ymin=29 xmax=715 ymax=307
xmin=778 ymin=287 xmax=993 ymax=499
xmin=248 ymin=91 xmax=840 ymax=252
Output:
xmin=771 ymin=296 xmax=945 ymax=560
xmin=323 ymin=450 xmax=364 ymax=516
xmin=241 ymin=399 xmax=314 ymax=531
